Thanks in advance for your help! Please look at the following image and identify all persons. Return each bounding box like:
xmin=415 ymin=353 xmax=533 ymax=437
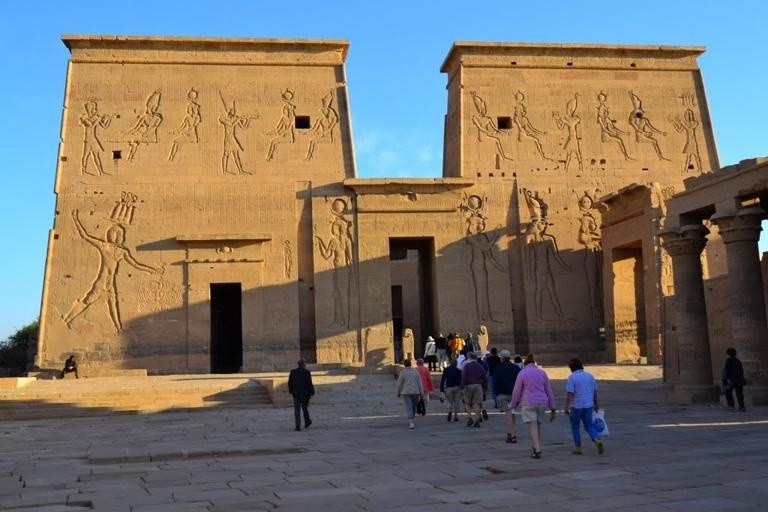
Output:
xmin=395 ymin=330 xmax=603 ymax=459
xmin=287 ymin=357 xmax=317 ymax=432
xmin=720 ymin=346 xmax=748 ymax=412
xmin=60 ymin=356 xmax=78 ymax=376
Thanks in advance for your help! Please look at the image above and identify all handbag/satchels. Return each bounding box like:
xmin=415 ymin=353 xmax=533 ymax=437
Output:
xmin=417 ymin=400 xmax=425 ymax=415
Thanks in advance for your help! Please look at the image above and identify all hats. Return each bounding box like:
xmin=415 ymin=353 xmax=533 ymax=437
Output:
xmin=498 ymin=350 xmax=511 ymax=358
xmin=524 ymin=354 xmax=535 ymax=365
xmin=427 ymin=336 xmax=435 ymax=341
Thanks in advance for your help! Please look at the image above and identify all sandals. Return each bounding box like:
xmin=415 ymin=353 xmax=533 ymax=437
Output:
xmin=506 ymin=433 xmax=542 ymax=459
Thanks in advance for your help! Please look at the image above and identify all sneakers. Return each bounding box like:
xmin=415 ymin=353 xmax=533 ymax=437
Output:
xmin=572 ymin=447 xmax=582 ymax=454
xmin=447 ymin=409 xmax=489 ymax=428
xmin=597 ymin=441 xmax=603 ymax=454
